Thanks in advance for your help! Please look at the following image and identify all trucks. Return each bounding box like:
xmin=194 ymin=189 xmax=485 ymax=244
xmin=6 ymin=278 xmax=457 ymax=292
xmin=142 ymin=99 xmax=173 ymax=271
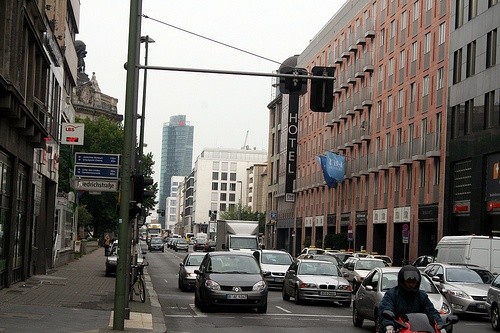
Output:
xmin=140 ymin=223 xmax=162 ymax=244
xmin=216 ymin=220 xmax=262 ymax=255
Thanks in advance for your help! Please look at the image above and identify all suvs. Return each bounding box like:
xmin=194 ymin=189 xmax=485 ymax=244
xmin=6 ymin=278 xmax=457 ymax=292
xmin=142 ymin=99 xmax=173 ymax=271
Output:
xmin=252 ymin=249 xmax=295 ymax=290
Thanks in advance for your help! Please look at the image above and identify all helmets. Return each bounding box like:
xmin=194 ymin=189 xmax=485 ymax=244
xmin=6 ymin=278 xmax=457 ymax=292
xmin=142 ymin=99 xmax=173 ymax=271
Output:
xmin=398 ymin=266 xmax=421 ymax=296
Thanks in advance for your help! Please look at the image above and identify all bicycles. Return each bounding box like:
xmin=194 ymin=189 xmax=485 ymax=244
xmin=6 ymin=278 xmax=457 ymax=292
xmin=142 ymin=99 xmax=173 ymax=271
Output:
xmin=130 ymin=261 xmax=149 ymax=303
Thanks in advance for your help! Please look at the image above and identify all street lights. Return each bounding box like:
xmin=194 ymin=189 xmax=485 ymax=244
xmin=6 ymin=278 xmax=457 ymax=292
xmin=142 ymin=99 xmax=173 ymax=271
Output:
xmin=237 ymin=180 xmax=242 ymax=219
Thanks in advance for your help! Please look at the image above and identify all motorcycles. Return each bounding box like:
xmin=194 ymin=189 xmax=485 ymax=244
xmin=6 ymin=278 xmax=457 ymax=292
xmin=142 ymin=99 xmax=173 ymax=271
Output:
xmin=379 ymin=309 xmax=459 ymax=333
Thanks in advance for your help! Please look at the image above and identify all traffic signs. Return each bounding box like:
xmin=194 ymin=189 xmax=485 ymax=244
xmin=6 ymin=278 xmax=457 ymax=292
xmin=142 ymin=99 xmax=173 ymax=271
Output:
xmin=74 ymin=152 xmax=120 ymax=179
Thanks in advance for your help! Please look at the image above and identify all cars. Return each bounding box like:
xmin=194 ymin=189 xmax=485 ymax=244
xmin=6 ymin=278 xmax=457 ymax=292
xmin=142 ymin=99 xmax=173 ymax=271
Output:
xmin=162 ymin=229 xmax=216 ymax=252
xmin=178 ymin=253 xmax=223 ymax=292
xmin=486 ymin=274 xmax=500 ymax=332
xmin=282 ymin=246 xmax=496 ymax=327
xmin=194 ymin=252 xmax=268 ymax=314
xmin=105 ymin=241 xmax=146 ymax=276
xmin=147 ymin=237 xmax=164 ymax=252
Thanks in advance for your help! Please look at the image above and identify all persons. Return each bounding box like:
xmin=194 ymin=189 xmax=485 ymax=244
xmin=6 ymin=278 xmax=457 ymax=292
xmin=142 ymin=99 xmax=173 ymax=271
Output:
xmin=104 ymin=236 xmax=112 ymax=256
xmin=183 ymin=236 xmax=191 ymax=245
xmin=377 ymin=265 xmax=443 ymax=333
xmin=422 ymin=257 xmax=429 ymax=266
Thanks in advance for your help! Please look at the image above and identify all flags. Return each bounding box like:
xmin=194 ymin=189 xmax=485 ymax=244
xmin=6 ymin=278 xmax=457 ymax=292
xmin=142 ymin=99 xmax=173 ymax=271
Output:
xmin=320 ymin=156 xmax=337 ymax=188
xmin=325 ymin=151 xmax=345 ymax=183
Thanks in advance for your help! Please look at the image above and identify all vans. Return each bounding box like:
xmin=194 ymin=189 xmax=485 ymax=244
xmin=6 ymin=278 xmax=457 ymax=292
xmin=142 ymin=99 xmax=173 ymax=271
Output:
xmin=432 ymin=234 xmax=500 ymax=277
